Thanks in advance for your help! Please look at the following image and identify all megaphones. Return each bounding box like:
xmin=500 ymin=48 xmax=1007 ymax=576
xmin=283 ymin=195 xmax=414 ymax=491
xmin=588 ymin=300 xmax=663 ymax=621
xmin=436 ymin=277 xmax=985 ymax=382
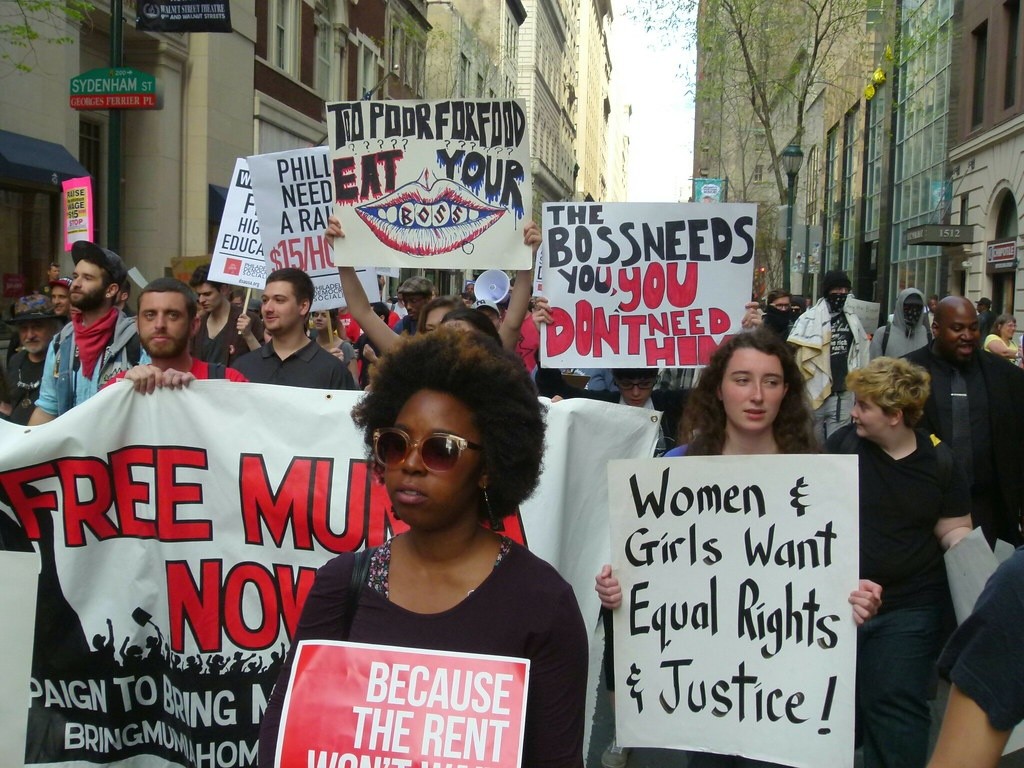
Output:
xmin=474 ymin=270 xmax=512 ymax=310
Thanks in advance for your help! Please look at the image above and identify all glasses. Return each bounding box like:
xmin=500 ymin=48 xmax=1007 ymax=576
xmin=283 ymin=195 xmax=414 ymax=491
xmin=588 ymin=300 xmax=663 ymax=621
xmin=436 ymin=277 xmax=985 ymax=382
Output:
xmin=775 ymin=302 xmax=791 ymax=310
xmin=372 ymin=427 xmax=488 ymax=476
xmin=613 ymin=377 xmax=660 ymax=391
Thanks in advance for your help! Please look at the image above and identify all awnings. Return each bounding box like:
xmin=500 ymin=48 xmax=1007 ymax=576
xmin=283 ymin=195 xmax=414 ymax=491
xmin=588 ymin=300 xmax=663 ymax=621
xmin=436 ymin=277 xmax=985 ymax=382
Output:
xmin=0 ymin=129 xmax=92 ymax=191
xmin=209 ymin=184 xmax=229 ymax=224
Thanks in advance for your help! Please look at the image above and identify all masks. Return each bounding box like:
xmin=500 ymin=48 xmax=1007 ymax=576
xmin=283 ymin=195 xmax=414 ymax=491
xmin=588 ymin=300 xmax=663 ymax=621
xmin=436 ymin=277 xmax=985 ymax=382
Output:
xmin=904 ymin=303 xmax=922 ymax=326
xmin=826 ymin=294 xmax=848 ymax=310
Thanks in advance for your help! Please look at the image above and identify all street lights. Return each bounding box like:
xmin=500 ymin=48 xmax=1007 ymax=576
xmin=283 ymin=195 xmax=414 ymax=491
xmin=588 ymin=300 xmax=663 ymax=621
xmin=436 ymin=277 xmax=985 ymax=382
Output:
xmin=780 ymin=140 xmax=804 ymax=291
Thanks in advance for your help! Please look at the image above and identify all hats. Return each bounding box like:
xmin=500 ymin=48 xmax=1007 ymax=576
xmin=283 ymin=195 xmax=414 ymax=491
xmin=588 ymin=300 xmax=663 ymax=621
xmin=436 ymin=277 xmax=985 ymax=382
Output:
xmin=974 ymin=297 xmax=992 ymax=307
xmin=471 ymin=299 xmax=501 ymax=317
xmin=48 ymin=276 xmax=73 ymax=292
xmin=822 ymin=270 xmax=853 ymax=296
xmin=398 ymin=276 xmax=433 ymax=295
xmin=3 ymin=294 xmax=68 ymax=325
xmin=71 ymin=239 xmax=128 ymax=287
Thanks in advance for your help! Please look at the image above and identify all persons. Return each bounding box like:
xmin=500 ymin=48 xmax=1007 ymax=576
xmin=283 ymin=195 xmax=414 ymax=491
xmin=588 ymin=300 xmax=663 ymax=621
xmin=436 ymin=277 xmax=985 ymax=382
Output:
xmin=595 ymin=271 xmax=1024 ymax=768
xmin=259 ymin=325 xmax=588 ymax=768
xmin=0 ymin=241 xmax=360 ymax=427
xmin=325 ymin=214 xmax=763 ymax=459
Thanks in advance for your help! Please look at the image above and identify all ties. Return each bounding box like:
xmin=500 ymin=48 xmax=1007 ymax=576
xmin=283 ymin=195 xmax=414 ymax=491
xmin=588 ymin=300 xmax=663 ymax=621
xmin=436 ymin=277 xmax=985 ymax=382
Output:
xmin=951 ymin=366 xmax=973 ymax=488
